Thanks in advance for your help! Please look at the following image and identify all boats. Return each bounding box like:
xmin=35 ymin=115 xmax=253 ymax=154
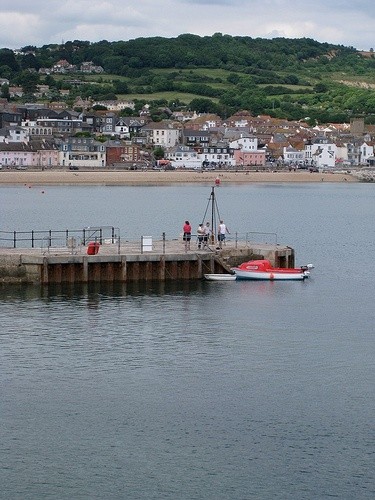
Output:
xmin=203 ymin=274 xmax=237 ymax=281
xmin=230 ymin=259 xmax=316 ymax=281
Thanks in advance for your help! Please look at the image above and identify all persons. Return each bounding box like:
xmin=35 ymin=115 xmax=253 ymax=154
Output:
xmin=197 ymin=222 xmax=210 ymax=249
xmin=183 ymin=221 xmax=192 ymax=252
xmin=216 ymin=220 xmax=231 ymax=249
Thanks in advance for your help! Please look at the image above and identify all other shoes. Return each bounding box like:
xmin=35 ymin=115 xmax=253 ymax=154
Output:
xmin=216 ymin=247 xmax=222 ymax=250
